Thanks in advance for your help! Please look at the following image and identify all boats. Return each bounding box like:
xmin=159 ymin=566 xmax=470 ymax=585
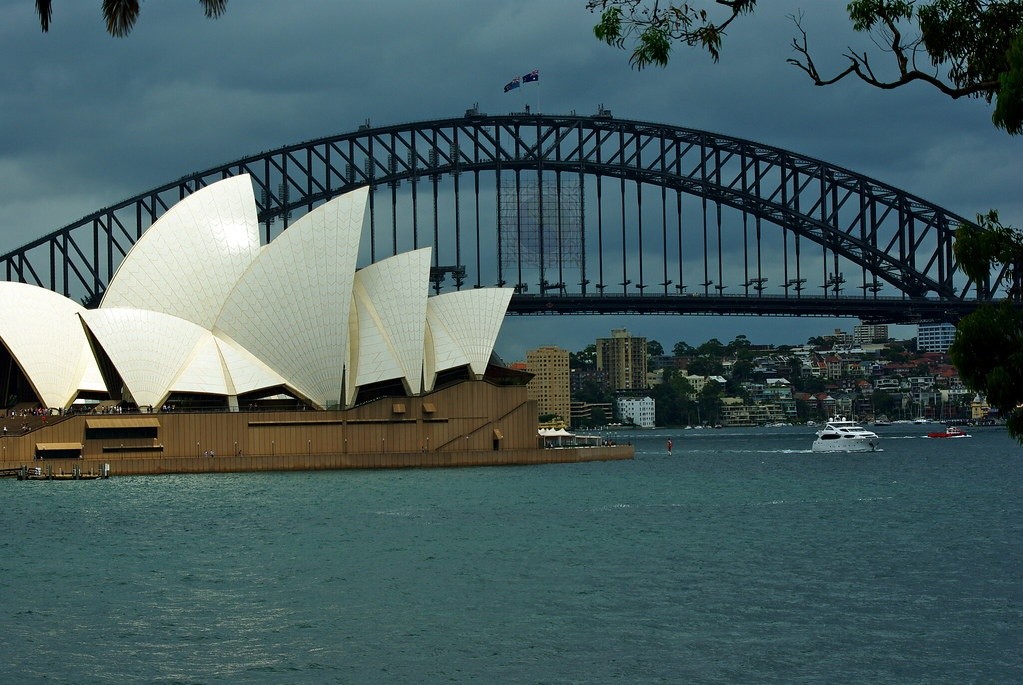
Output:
xmin=694 ymin=426 xmax=704 ymax=430
xmin=914 ymin=417 xmax=930 ymax=424
xmin=812 ymin=420 xmax=881 ymax=452
xmin=684 ymin=426 xmax=691 ymax=430
xmin=715 ymin=425 xmax=722 ymax=429
xmin=928 ymin=427 xmax=966 ymax=438
xmin=874 ymin=420 xmax=891 ymax=426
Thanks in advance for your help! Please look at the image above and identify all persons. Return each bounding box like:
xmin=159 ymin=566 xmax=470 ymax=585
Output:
xmin=239 ymin=450 xmax=241 ymax=455
xmin=604 ymin=439 xmax=612 ymax=446
xmin=40 ymin=456 xmax=43 ymax=461
xmin=4 ymin=426 xmax=7 ymax=435
xmin=205 ymin=450 xmax=214 ymax=457
xmin=12 ymin=404 xmax=123 ymax=433
xmin=33 ymin=455 xmax=37 ymax=461
xmin=666 ymin=438 xmax=672 ymax=455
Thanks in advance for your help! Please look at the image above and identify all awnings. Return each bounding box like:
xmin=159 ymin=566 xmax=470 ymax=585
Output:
xmin=85 ymin=418 xmax=161 ymax=428
xmin=36 ymin=442 xmax=83 ymax=450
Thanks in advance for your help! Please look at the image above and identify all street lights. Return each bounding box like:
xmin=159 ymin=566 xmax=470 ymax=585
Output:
xmin=615 ymin=432 xmax=617 ymax=443
xmin=610 ymin=432 xmax=611 ymax=439
xmin=606 ymin=432 xmax=608 ymax=439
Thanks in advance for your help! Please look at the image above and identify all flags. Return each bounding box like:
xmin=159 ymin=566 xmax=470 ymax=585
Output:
xmin=504 ymin=77 xmax=520 ymax=93
xmin=522 ymin=70 xmax=538 ymax=83
